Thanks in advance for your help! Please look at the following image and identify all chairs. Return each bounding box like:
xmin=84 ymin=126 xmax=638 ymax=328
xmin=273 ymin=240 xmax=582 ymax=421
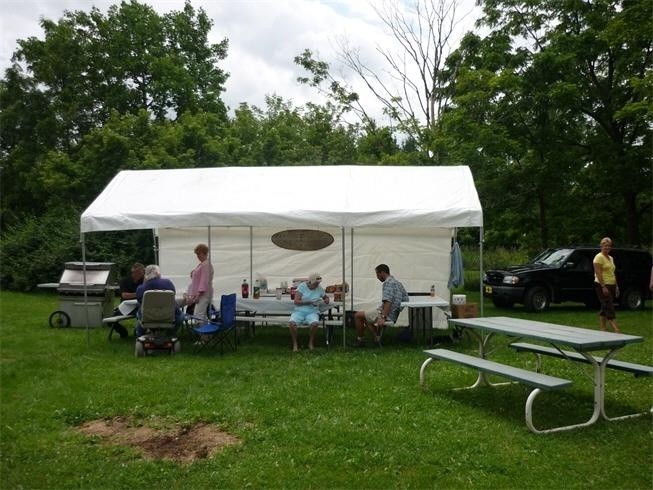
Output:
xmin=191 ymin=292 xmax=236 ymax=356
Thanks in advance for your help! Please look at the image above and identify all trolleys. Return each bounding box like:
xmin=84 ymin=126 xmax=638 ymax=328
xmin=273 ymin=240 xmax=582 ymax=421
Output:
xmin=48 ymin=260 xmax=117 ymax=330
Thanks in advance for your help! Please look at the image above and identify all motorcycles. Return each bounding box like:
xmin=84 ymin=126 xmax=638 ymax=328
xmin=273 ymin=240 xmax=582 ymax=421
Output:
xmin=132 ymin=290 xmax=182 ymax=355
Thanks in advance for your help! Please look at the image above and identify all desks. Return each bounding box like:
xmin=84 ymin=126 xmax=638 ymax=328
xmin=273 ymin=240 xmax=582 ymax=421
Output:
xmin=401 ymin=294 xmax=449 ymax=348
xmin=118 ymin=293 xmax=192 ymax=317
xmin=236 ymin=292 xmax=343 ymax=342
xmin=448 ymin=316 xmax=644 ymax=434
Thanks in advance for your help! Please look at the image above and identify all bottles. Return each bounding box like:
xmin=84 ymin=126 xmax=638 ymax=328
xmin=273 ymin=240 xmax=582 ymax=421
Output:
xmin=279 ymin=280 xmax=288 ymax=291
xmin=253 ymin=280 xmax=260 ymax=300
xmin=260 ymin=280 xmax=267 ymax=297
xmin=241 ymin=279 xmax=249 ymax=299
xmin=430 ymin=284 xmax=434 ymax=298
xmin=290 ymin=283 xmax=296 ymax=300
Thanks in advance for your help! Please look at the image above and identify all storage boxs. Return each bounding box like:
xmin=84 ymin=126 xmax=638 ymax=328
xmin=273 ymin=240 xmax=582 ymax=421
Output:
xmin=451 ymin=303 xmax=477 ymax=319
xmin=452 ymin=294 xmax=467 ymax=304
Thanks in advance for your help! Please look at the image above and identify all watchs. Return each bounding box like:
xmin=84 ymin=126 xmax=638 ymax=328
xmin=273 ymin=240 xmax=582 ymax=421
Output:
xmin=381 ymin=315 xmax=386 ymax=318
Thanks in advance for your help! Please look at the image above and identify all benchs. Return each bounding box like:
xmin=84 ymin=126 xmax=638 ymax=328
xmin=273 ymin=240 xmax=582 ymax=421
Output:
xmin=509 ymin=342 xmax=653 ymax=422
xmin=103 ymin=314 xmax=136 ymax=342
xmin=235 ymin=316 xmax=343 ymax=352
xmin=374 ymin=320 xmax=394 ymax=349
xmin=420 ymin=348 xmax=573 ymax=435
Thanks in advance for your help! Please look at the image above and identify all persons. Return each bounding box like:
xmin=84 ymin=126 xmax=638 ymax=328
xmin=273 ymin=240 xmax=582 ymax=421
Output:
xmin=136 ymin=265 xmax=185 ymax=338
xmin=649 ymin=267 xmax=653 ymax=290
xmin=108 ymin=263 xmax=145 ymax=339
xmin=593 ymin=238 xmax=620 ymax=334
xmin=187 ymin=271 xmax=216 ymax=320
xmin=289 ymin=273 xmax=329 ymax=352
xmin=191 ymin=244 xmax=214 ymax=345
xmin=352 ymin=264 xmax=409 ymax=348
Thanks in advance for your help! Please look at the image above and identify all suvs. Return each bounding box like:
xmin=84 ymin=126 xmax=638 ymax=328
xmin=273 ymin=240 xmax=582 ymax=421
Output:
xmin=480 ymin=245 xmax=652 ymax=312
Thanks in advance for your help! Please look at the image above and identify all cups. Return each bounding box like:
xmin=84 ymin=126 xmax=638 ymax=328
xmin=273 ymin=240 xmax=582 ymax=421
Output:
xmin=276 ymin=288 xmax=282 ymax=300
